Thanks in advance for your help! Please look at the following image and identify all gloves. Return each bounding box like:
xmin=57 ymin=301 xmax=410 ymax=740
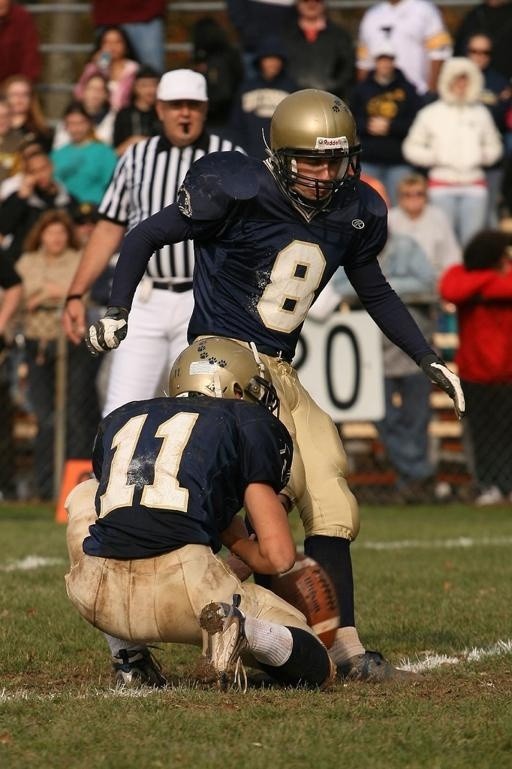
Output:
xmin=83 ymin=306 xmax=129 ymax=356
xmin=419 ymin=353 xmax=466 ymax=422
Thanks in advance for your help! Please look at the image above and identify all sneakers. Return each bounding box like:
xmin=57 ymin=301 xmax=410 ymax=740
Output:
xmin=109 ymin=644 xmax=169 ymax=691
xmin=475 ymin=484 xmax=504 ymax=508
xmin=197 ymin=601 xmax=250 ymax=693
xmin=396 ymin=478 xmax=454 ymax=503
xmin=331 ymin=650 xmax=427 ymax=686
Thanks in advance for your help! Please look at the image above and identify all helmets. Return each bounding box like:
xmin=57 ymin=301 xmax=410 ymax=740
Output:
xmin=267 ymin=88 xmax=362 ymax=213
xmin=167 ymin=335 xmax=281 ymax=415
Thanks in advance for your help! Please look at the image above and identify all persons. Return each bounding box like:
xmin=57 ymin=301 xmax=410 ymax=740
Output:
xmin=3 ymin=0 xmax=511 ymax=515
xmin=59 ymin=334 xmax=340 ymax=691
xmin=83 ymin=87 xmax=467 ymax=681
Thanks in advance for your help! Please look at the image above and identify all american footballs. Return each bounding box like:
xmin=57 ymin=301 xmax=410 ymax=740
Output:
xmin=268 ymin=550 xmax=341 ymax=650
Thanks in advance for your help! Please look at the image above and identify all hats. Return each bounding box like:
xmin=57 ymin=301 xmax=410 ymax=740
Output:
xmin=374 ymin=46 xmax=395 ymax=59
xmin=154 ymin=68 xmax=208 ymax=102
